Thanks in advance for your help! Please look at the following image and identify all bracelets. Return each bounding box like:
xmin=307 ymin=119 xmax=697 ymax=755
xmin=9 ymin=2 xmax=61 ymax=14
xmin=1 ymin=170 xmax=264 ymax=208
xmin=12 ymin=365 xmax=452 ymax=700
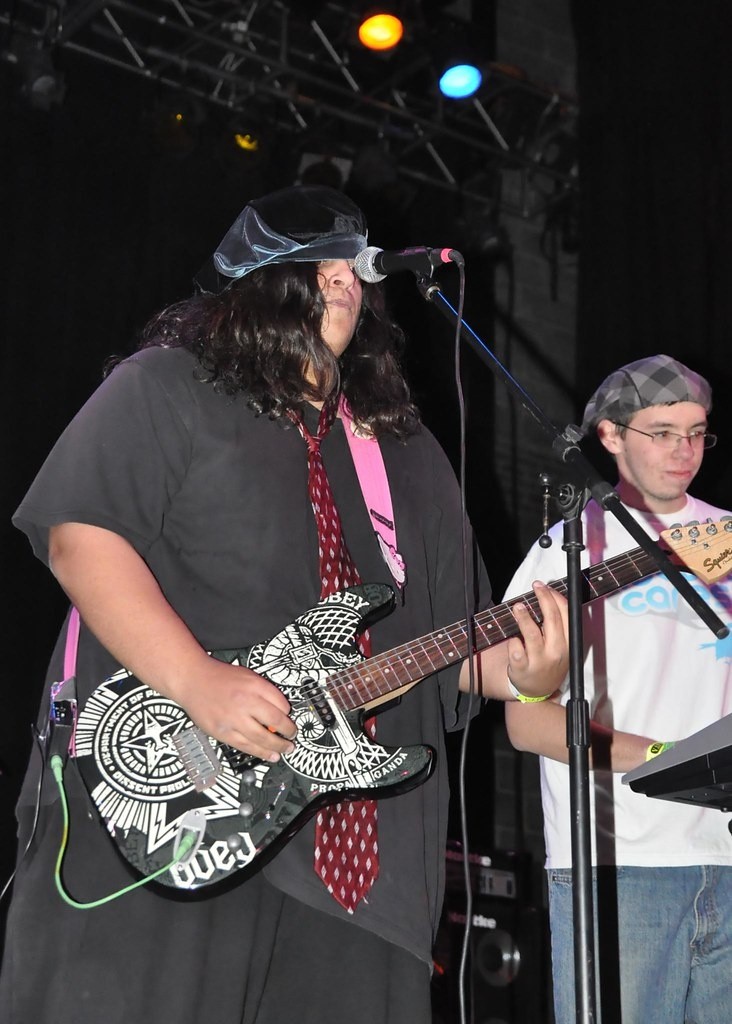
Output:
xmin=646 ymin=742 xmax=664 ymax=762
xmin=506 ymin=677 xmax=554 ymax=704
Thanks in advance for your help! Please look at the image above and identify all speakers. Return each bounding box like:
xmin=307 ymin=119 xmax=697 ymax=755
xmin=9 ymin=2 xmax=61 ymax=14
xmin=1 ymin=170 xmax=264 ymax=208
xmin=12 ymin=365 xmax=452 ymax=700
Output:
xmin=431 ymin=846 xmax=544 ymax=1024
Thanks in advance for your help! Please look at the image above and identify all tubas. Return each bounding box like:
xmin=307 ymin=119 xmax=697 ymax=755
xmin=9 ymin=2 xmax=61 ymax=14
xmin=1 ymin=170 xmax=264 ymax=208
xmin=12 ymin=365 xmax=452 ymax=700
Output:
xmin=71 ymin=517 xmax=732 ymax=903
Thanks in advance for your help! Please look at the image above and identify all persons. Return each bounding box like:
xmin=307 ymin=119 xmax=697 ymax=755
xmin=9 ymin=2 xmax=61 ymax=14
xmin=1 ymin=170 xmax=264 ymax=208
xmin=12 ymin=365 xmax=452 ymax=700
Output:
xmin=1 ymin=186 xmax=568 ymax=1024
xmin=500 ymin=354 xmax=732 ymax=1024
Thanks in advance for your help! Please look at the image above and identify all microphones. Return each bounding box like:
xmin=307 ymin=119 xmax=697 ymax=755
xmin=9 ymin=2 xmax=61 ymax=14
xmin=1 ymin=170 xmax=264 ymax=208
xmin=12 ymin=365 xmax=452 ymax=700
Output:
xmin=354 ymin=245 xmax=456 ymax=284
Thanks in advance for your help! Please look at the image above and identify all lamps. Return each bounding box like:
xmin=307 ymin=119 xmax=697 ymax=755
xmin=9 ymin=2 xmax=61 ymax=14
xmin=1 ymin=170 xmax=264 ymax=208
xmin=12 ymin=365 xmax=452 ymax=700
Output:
xmin=432 ymin=11 xmax=482 ymax=100
xmin=354 ymin=0 xmax=404 ymax=52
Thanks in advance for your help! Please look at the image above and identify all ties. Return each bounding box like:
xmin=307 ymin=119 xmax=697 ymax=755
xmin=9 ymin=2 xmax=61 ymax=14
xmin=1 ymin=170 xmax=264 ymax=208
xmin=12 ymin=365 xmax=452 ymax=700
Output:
xmin=283 ymin=402 xmax=379 ymax=915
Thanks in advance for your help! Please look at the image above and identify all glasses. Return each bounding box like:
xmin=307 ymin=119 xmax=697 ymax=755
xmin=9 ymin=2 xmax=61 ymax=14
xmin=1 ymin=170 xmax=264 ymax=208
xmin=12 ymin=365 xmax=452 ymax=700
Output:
xmin=613 ymin=422 xmax=717 ymax=450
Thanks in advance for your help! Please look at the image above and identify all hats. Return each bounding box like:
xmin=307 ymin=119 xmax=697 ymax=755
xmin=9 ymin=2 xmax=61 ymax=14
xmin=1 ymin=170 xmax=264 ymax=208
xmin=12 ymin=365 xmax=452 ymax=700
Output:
xmin=582 ymin=356 xmax=712 ymax=429
xmin=213 ymin=185 xmax=368 ymax=279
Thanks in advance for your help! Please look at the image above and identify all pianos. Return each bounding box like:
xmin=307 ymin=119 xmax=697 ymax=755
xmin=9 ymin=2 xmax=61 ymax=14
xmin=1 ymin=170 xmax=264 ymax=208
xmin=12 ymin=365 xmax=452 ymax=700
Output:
xmin=619 ymin=711 xmax=732 ymax=814
